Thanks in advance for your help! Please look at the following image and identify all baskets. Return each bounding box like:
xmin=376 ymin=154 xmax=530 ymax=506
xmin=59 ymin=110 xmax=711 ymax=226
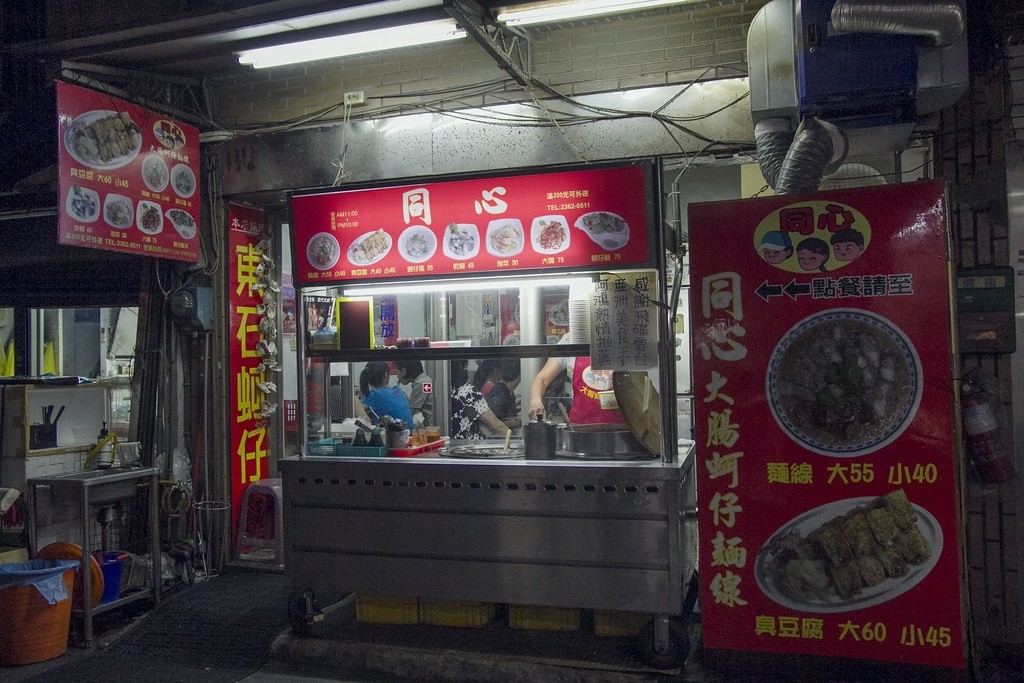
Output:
xmin=337 ymin=444 xmax=389 ymax=457
xmin=307 ymin=437 xmax=355 ymax=456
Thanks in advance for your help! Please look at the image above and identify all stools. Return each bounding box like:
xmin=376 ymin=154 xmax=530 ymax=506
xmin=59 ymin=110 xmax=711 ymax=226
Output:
xmin=237 ymin=476 xmax=287 ymax=572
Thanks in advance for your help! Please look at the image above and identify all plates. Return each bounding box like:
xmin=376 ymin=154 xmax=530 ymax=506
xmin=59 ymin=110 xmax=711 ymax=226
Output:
xmin=502 ymin=298 xmax=568 ymax=344
xmin=64 ymin=109 xmax=196 ymax=240
xmin=307 ymin=211 xmax=630 ymax=271
xmin=765 ymin=306 xmax=925 ymax=459
xmin=754 ymin=496 xmax=943 ymax=613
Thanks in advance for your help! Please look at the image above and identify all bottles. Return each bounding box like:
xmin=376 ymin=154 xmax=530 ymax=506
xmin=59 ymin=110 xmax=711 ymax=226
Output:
xmin=368 ymin=428 xmax=384 ymax=447
xmin=352 ymin=428 xmax=367 ymax=446
xmin=961 ymin=369 xmax=1014 ymax=481
xmin=98 ymin=422 xmax=113 ymax=469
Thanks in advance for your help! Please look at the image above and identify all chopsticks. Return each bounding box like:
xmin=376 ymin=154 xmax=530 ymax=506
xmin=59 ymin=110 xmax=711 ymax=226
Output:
xmin=41 ymin=404 xmax=65 ymax=425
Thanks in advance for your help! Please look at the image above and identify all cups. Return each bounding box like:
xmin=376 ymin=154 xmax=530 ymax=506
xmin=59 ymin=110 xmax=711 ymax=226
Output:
xmin=522 ymin=413 xmax=558 ymax=460
xmin=412 ymin=429 xmax=427 ymax=448
xmin=425 ymin=426 xmax=441 ymax=443
xmin=568 ymin=278 xmax=591 ymax=344
xmin=390 ymin=429 xmax=410 ymax=448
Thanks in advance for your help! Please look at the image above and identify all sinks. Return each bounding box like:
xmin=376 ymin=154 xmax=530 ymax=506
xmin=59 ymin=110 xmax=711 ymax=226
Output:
xmin=49 ymin=467 xmax=142 ymax=505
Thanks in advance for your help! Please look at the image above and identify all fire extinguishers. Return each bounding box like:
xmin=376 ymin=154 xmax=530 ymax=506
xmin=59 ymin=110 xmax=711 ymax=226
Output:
xmin=959 ymin=366 xmax=1017 ymax=483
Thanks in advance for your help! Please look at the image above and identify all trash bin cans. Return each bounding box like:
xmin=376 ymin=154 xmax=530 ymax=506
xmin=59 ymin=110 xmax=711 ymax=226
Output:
xmin=0 ymin=558 xmax=80 ymax=666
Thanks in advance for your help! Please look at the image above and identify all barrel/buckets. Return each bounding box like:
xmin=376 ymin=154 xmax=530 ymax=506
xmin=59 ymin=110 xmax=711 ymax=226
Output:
xmin=91 ymin=551 xmax=130 ymax=605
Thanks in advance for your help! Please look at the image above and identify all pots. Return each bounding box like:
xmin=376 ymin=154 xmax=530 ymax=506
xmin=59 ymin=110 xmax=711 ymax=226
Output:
xmin=438 ymin=444 xmax=524 ymax=458
xmin=562 ymin=424 xmax=647 ymax=456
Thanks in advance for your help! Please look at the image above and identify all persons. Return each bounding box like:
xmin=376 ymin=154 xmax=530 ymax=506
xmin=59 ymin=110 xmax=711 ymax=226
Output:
xmin=329 ymin=375 xmax=369 ymax=424
xmin=358 ymin=360 xmax=415 ymax=432
xmin=450 ymin=360 xmax=513 ymax=438
xmin=488 ymin=359 xmax=521 ymax=434
xmin=528 ymin=331 xmax=630 ymax=425
xmin=472 ymin=358 xmax=501 ymax=396
xmin=394 ymin=358 xmax=436 ymax=427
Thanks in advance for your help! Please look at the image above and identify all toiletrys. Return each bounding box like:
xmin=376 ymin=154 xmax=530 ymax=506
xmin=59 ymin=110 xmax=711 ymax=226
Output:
xmin=93 ymin=421 xmax=118 ymax=469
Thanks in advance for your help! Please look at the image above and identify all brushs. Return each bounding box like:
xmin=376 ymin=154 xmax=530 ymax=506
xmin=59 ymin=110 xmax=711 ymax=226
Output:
xmin=97 ymin=550 xmax=129 ymax=566
